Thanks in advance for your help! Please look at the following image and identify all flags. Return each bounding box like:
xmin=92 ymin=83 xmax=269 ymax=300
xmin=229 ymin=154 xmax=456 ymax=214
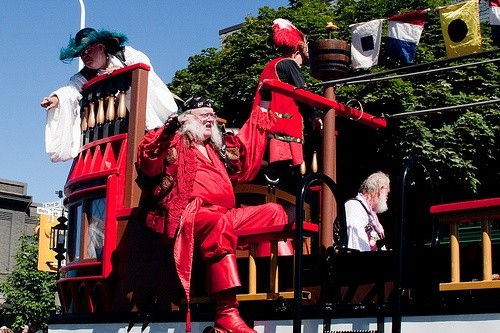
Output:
xmin=350 ymin=0 xmax=500 ymax=70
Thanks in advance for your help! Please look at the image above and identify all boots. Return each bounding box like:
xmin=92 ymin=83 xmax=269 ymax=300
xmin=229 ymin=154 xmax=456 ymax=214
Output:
xmin=213 ymin=293 xmax=257 ymax=333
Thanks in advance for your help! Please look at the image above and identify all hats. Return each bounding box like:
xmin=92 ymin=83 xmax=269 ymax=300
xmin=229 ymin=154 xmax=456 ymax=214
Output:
xmin=272 ymin=18 xmax=310 ymax=57
xmin=59 ymin=27 xmax=128 ymax=61
xmin=181 ymin=96 xmax=214 ymax=112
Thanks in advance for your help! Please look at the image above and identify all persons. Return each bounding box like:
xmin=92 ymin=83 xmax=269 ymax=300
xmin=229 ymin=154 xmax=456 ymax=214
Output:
xmin=259 ymin=19 xmax=323 ymax=222
xmin=40 ymin=28 xmax=178 ymax=161
xmin=344 ymin=171 xmax=391 ymax=249
xmin=133 ymin=97 xmax=288 ymax=333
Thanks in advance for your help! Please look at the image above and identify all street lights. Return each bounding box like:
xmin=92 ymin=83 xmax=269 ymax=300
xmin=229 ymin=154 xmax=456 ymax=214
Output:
xmin=45 ymin=209 xmax=69 ymax=316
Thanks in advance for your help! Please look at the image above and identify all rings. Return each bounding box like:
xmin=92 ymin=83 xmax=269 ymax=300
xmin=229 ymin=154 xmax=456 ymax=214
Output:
xmin=45 ymin=98 xmax=50 ymax=102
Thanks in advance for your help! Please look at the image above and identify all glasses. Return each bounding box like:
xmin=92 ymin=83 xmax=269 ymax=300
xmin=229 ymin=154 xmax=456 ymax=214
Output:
xmin=194 ymin=114 xmax=217 ymax=125
xmin=78 ymin=48 xmax=93 ymax=57
